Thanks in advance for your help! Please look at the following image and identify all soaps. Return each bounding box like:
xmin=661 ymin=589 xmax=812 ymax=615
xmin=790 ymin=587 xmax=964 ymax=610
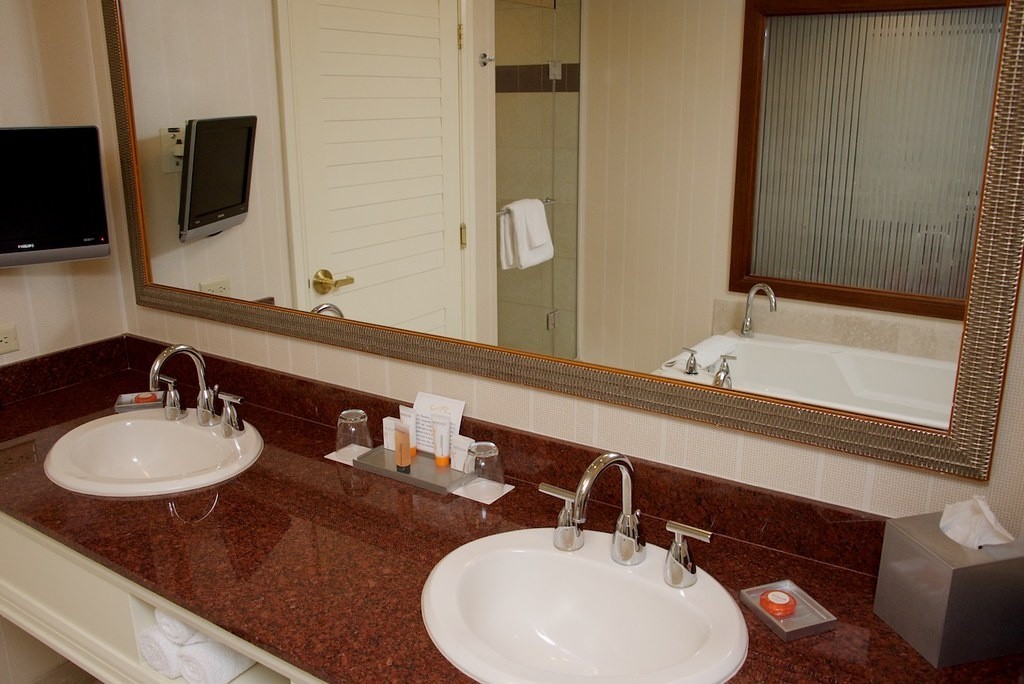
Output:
xmin=134 ymin=392 xmax=156 ymax=404
xmin=759 ymin=590 xmax=797 ymax=618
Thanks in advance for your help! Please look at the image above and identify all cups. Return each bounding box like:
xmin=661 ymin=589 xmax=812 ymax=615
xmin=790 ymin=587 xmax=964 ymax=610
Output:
xmin=462 ymin=440 xmax=505 ymax=500
xmin=335 ymin=409 xmax=373 ymax=462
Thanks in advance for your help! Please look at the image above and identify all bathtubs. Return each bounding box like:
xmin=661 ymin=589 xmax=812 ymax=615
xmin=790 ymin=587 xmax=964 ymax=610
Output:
xmin=651 ymin=328 xmax=960 ymax=430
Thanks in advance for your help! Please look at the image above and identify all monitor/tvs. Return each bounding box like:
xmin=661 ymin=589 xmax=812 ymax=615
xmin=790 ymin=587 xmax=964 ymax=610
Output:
xmin=0 ymin=125 xmax=113 ymax=268
xmin=177 ymin=114 xmax=258 ymax=243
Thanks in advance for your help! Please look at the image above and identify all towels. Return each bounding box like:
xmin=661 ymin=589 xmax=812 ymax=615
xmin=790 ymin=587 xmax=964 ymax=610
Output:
xmin=501 ymin=198 xmax=556 ymax=271
xmin=137 ymin=607 xmax=258 ymax=684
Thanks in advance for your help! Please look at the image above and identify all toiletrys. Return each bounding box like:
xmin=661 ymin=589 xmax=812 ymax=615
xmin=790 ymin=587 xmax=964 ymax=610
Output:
xmin=382 ymin=404 xmax=475 ymax=474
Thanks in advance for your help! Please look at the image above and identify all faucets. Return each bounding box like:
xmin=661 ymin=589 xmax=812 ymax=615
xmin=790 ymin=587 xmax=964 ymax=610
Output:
xmin=149 ymin=344 xmax=219 ymax=428
xmin=570 ymin=450 xmax=647 ymax=566
xmin=712 ymin=354 xmax=734 ymax=391
xmin=742 ymin=283 xmax=776 ymax=337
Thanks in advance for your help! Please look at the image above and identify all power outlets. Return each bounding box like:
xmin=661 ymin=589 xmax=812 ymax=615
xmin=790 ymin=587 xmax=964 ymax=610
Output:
xmin=200 ymin=277 xmax=233 ymax=297
xmin=0 ymin=325 xmax=19 ymax=355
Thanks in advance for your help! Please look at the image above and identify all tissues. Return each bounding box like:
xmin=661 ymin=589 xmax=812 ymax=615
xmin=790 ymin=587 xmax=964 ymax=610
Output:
xmin=872 ymin=496 xmax=1024 ymax=669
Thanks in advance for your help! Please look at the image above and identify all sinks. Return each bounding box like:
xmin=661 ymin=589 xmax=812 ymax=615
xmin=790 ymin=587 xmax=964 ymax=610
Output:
xmin=420 ymin=526 xmax=750 ymax=684
xmin=42 ymin=406 xmax=264 ymax=500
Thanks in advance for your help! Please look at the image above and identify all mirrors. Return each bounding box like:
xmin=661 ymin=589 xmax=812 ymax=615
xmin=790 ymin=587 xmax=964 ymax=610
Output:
xmin=119 ymin=0 xmax=1009 ymax=433
